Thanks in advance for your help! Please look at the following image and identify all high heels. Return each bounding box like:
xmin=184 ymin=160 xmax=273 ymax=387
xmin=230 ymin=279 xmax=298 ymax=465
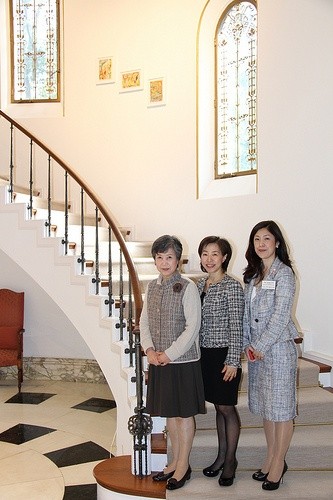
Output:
xmin=203 ymin=462 xmax=224 ymax=477
xmin=167 ymin=464 xmax=192 ymax=490
xmin=262 ymin=460 xmax=288 ymax=490
xmin=219 ymin=462 xmax=238 ymax=486
xmin=253 ymin=468 xmax=269 ymax=481
xmin=152 ymin=468 xmax=175 ymax=481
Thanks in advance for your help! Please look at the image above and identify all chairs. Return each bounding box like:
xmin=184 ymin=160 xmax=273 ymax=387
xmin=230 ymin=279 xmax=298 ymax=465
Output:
xmin=0 ymin=289 xmax=25 ymax=393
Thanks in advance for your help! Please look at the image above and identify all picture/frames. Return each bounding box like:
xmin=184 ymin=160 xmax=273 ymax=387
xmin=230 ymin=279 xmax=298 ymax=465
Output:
xmin=146 ymin=77 xmax=166 ymax=108
xmin=118 ymin=68 xmax=144 ymax=93
xmin=96 ymin=55 xmax=116 ymax=85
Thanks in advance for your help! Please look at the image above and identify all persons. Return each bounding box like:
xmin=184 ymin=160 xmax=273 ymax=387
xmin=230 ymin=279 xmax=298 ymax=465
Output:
xmin=242 ymin=220 xmax=299 ymax=490
xmin=140 ymin=234 xmax=203 ymax=490
xmin=195 ymin=236 xmax=245 ymax=488
